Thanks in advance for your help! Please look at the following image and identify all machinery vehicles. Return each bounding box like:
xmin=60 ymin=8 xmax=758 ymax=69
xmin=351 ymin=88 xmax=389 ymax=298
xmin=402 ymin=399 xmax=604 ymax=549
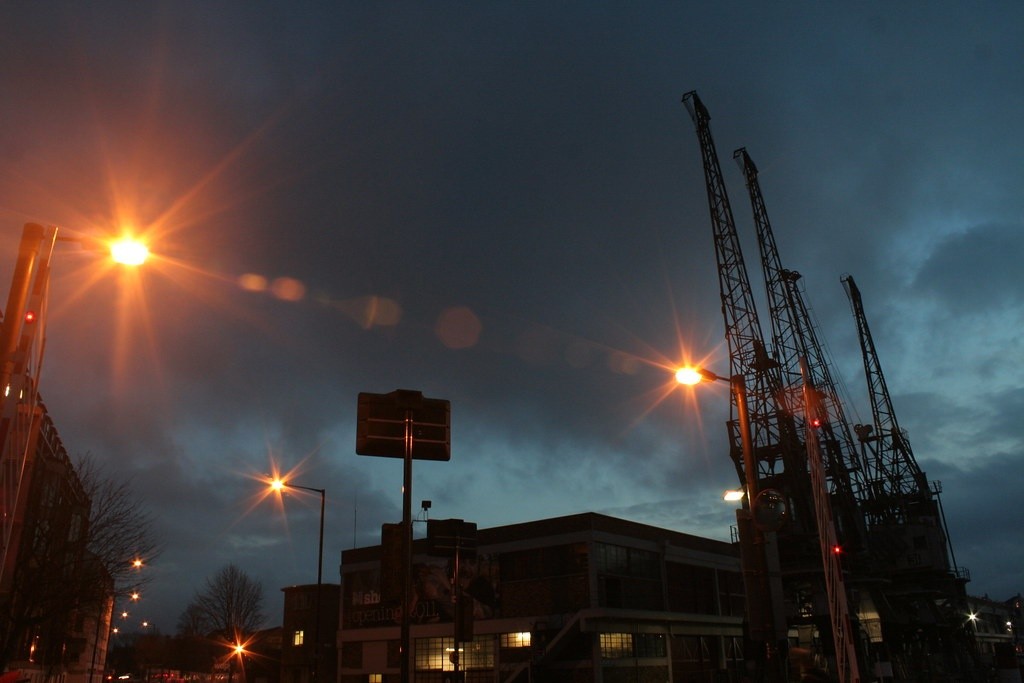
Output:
xmin=680 ymin=83 xmax=976 ymax=683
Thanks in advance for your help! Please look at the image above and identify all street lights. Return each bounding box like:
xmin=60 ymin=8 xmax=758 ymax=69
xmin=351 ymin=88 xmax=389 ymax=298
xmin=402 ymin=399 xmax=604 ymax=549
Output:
xmin=1 ymin=222 xmax=151 ymax=431
xmin=227 ymin=641 xmax=248 ymax=683
xmin=262 ymin=471 xmax=328 ymax=683
xmin=674 ymin=365 xmax=786 ymax=683
xmin=90 ymin=556 xmax=143 ymax=683
